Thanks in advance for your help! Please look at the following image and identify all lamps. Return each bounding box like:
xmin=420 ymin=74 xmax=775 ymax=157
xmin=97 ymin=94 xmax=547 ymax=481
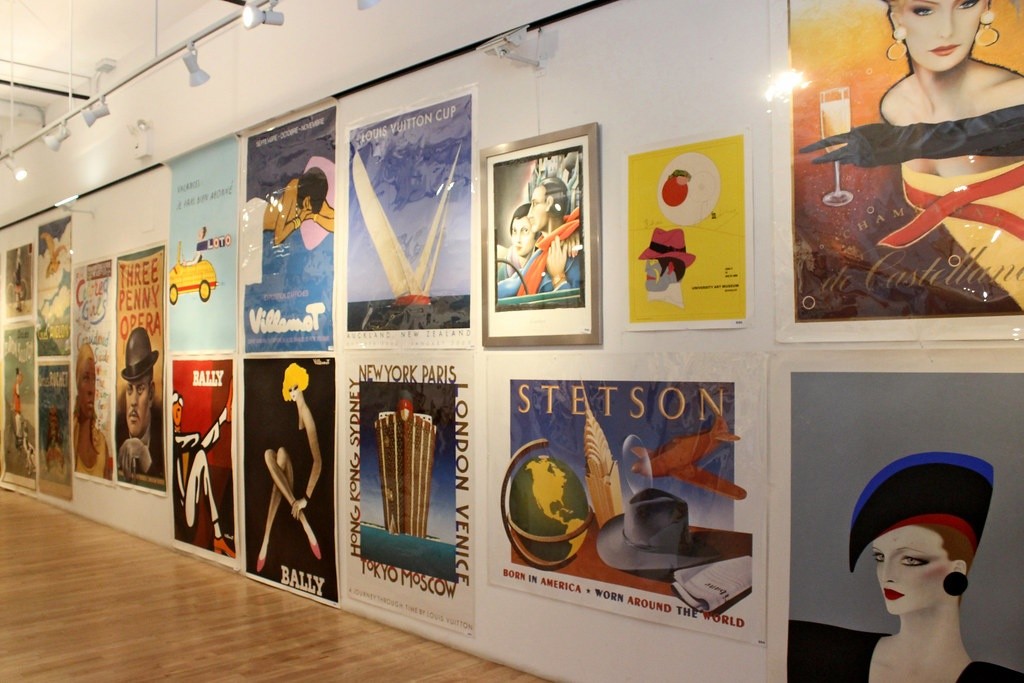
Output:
xmin=242 ymin=0 xmax=285 ymax=29
xmin=6 ymin=150 xmax=29 ymax=182
xmin=125 ymin=118 xmax=154 ymax=160
xmin=476 ymin=26 xmax=547 ymax=74
xmin=55 ymin=195 xmax=95 ymax=214
xmin=181 ymin=44 xmax=210 ymax=89
xmin=43 ymin=122 xmax=72 ymax=153
xmin=82 ymin=96 xmax=111 ymax=128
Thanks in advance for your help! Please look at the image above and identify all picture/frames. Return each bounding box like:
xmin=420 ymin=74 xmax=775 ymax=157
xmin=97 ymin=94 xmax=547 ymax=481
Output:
xmin=479 ymin=122 xmax=605 ymax=350
xmin=765 ymin=345 xmax=1024 ymax=683
xmin=773 ymin=0 xmax=1024 ymax=342
